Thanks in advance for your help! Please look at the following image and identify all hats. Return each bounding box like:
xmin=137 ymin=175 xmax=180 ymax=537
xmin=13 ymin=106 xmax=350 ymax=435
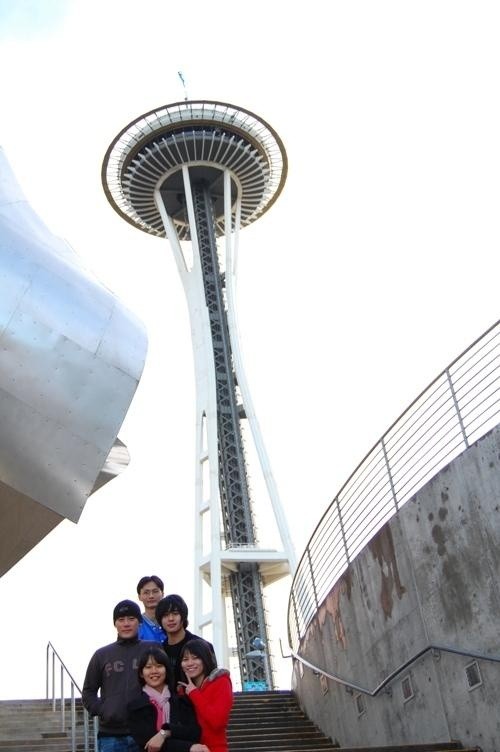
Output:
xmin=114 ymin=600 xmax=143 ymax=624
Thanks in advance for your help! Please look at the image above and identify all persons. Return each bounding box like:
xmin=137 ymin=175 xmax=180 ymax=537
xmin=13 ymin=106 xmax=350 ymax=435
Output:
xmin=137 ymin=575 xmax=168 ymax=643
xmin=177 ymin=639 xmax=233 ymax=752
xmin=81 ymin=599 xmax=161 ymax=752
xmin=128 ymin=647 xmax=210 ymax=752
xmin=155 ymin=594 xmax=218 ymax=689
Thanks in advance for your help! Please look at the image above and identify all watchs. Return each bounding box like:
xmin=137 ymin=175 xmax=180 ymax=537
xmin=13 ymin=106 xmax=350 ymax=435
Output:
xmin=160 ymin=729 xmax=167 ymax=738
